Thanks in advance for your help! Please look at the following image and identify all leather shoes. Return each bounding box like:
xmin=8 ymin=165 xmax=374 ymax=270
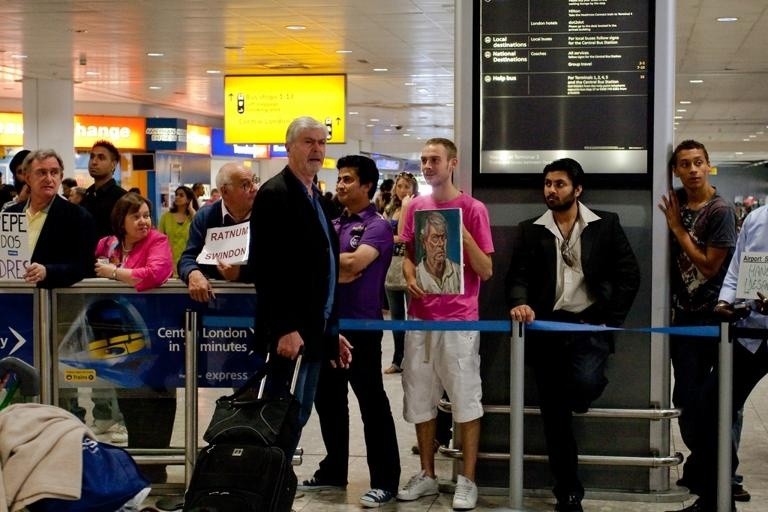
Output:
xmin=665 ymin=494 xmax=738 ymax=512
xmin=555 ymin=497 xmax=584 ymax=512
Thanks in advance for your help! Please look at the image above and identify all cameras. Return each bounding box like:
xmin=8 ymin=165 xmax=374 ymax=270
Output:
xmin=728 ymin=298 xmax=751 ymax=319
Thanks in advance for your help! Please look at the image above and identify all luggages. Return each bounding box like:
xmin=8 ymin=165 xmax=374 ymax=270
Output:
xmin=182 ymin=440 xmax=299 ymax=511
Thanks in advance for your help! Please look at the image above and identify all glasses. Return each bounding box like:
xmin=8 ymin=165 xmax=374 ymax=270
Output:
xmin=396 ymin=172 xmax=415 ymax=178
xmin=428 ymin=232 xmax=448 ymax=241
xmin=224 ymin=176 xmax=260 ymax=191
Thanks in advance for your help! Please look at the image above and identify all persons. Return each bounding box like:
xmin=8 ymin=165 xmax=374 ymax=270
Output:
xmin=656 ymin=137 xmax=752 ymax=503
xmin=245 ymin=114 xmax=354 ymax=466
xmin=664 ymin=202 xmax=767 ymax=512
xmin=499 ymin=156 xmax=640 ymax=511
xmin=736 ymin=195 xmax=760 ymax=234
xmin=2 ymin=137 xmax=495 ymax=510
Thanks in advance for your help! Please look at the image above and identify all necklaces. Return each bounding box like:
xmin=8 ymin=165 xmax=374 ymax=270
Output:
xmin=551 ymin=208 xmax=580 ymax=245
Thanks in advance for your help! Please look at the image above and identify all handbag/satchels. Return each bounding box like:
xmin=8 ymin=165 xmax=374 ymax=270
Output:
xmin=202 ymin=395 xmax=302 ymax=462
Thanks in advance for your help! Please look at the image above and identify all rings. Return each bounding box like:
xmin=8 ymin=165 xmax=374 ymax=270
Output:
xmin=762 ymin=298 xmax=767 ymax=304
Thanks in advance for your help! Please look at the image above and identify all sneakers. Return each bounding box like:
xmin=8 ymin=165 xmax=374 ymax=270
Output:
xmin=360 ymin=487 xmax=396 ymax=508
xmin=396 ymin=470 xmax=438 ymax=501
xmin=732 ymin=482 xmax=751 ymax=501
xmin=296 ymin=471 xmax=348 ymax=490
xmin=385 ymin=366 xmax=403 ymax=373
xmin=452 ymin=474 xmax=478 ymax=509
